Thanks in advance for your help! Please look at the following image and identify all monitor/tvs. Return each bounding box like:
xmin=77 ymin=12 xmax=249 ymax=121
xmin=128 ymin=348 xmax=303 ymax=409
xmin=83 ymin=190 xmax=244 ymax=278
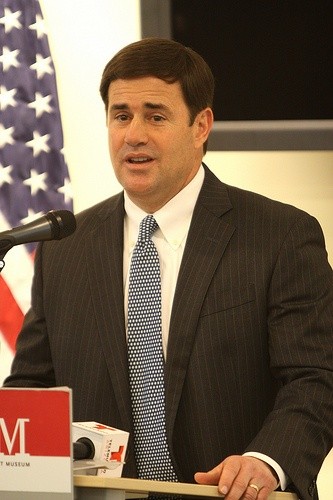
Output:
xmin=139 ymin=0 xmax=333 ymax=151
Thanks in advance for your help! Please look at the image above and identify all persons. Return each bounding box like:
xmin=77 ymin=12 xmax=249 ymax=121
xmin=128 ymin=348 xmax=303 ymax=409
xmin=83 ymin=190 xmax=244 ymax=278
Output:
xmin=1 ymin=38 xmax=332 ymax=500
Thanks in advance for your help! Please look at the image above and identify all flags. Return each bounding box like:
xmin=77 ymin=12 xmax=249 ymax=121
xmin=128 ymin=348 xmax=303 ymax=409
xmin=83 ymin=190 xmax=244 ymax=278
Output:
xmin=0 ymin=0 xmax=76 ymax=360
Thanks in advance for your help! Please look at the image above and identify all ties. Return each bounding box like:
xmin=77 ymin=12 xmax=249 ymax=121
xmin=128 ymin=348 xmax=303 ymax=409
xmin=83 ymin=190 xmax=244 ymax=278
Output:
xmin=125 ymin=216 xmax=178 ymax=481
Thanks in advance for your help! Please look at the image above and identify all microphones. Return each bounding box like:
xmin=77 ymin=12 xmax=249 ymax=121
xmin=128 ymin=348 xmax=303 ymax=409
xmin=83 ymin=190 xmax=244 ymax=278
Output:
xmin=0 ymin=209 xmax=77 ymax=252
xmin=72 ymin=421 xmax=130 ymax=477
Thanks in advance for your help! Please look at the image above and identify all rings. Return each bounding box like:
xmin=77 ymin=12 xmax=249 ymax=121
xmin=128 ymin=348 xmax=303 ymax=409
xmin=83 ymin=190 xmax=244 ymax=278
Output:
xmin=249 ymin=484 xmax=259 ymax=493
